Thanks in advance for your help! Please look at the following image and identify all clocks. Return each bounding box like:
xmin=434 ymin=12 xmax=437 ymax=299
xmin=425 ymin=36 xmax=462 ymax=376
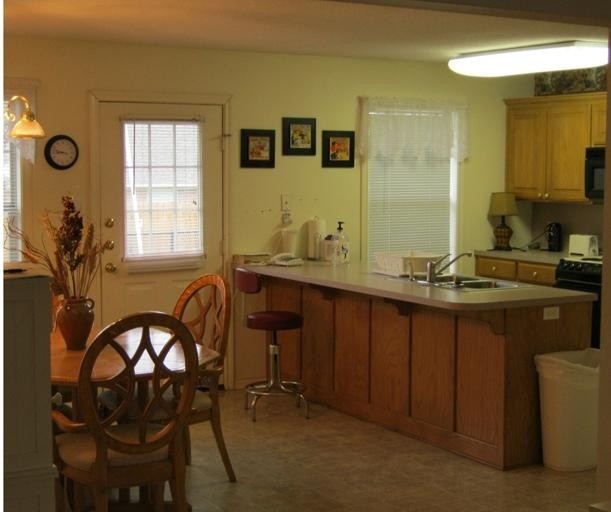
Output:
xmin=44 ymin=134 xmax=80 ymax=169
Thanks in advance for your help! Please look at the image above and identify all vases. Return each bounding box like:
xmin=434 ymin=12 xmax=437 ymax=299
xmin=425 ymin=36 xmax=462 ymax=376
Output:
xmin=54 ymin=296 xmax=99 ymax=354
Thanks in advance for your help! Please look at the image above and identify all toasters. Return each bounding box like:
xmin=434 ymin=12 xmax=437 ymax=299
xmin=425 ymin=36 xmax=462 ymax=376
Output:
xmin=569 ymin=233 xmax=599 ymax=257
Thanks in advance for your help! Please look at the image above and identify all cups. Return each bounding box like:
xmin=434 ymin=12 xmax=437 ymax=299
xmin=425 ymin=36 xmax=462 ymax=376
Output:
xmin=281 ymin=229 xmax=297 ymax=259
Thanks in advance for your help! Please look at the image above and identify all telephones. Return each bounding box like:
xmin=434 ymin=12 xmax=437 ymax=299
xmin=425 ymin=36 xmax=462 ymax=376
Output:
xmin=270 ymin=252 xmax=304 ymax=266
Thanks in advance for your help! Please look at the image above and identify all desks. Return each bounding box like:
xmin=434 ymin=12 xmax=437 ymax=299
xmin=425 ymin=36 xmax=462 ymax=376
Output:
xmin=46 ymin=323 xmax=224 ymax=510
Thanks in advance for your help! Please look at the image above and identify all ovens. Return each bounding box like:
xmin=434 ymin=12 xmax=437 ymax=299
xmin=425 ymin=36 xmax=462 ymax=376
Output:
xmin=551 ymin=274 xmax=600 ymax=349
xmin=585 ymin=147 xmax=604 ymax=203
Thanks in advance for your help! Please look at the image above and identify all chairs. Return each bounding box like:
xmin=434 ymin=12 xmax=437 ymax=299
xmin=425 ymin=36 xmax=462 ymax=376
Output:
xmin=51 ymin=311 xmax=200 ymax=510
xmin=236 ymin=262 xmax=313 ymax=420
xmin=53 ymin=385 xmax=101 ymax=484
xmin=99 ymin=272 xmax=245 ymax=492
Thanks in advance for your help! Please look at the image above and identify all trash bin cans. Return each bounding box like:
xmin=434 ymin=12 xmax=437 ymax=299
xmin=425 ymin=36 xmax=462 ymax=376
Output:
xmin=534 ymin=349 xmax=599 ymax=472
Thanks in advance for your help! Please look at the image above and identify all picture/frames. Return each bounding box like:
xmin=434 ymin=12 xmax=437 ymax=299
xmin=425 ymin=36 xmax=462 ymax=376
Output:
xmin=282 ymin=115 xmax=318 ymax=155
xmin=321 ymin=128 xmax=358 ymax=169
xmin=238 ymin=126 xmax=277 ymax=171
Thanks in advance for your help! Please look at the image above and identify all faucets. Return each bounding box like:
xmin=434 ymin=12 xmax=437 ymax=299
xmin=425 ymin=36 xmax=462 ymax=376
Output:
xmin=425 ymin=254 xmax=451 ymax=282
xmin=427 ymin=252 xmax=472 ymax=283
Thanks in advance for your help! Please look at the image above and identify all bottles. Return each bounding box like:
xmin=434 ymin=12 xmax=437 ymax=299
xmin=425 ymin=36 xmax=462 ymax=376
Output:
xmin=307 ymin=216 xmax=349 ymax=260
xmin=548 ymin=222 xmax=562 ymax=252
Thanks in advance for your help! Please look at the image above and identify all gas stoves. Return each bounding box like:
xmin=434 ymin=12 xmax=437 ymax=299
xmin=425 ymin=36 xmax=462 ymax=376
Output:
xmin=555 ymin=255 xmax=602 ymax=283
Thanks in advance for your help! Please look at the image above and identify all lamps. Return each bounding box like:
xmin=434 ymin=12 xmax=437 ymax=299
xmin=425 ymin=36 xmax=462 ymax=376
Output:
xmin=4 ymin=92 xmax=47 ymax=141
xmin=448 ymin=35 xmax=611 ymax=78
xmin=488 ymin=193 xmax=522 ymax=252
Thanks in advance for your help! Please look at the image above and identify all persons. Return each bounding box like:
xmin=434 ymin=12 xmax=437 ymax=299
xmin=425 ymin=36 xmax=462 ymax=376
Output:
xmin=331 ymin=141 xmax=341 ymax=159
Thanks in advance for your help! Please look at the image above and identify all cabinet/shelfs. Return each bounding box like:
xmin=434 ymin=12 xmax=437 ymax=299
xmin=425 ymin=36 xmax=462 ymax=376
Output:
xmin=589 ymin=88 xmax=611 ymax=148
xmin=472 ymin=255 xmax=559 ymax=286
xmin=3 ymin=258 xmax=58 ymax=511
xmin=503 ymin=91 xmax=588 ymax=206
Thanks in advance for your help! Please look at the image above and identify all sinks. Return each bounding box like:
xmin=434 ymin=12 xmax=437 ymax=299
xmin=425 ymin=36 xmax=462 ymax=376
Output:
xmin=444 ymin=280 xmax=524 ymax=292
xmin=415 ymin=274 xmax=480 ymax=286
